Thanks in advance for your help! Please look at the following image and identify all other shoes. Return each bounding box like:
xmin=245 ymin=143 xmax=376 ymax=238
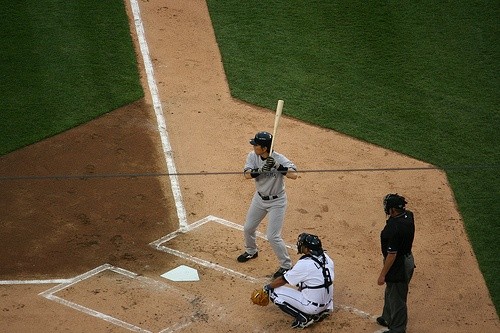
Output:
xmin=291 ymin=318 xmax=314 ymax=329
xmin=274 ymin=267 xmax=292 ymax=279
xmin=375 ymin=317 xmax=389 ymax=327
xmin=237 ymin=251 xmax=258 ymax=262
xmin=314 ymin=312 xmax=330 ymax=322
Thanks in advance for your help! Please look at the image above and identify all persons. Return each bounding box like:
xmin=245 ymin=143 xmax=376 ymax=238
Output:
xmin=375 ymin=193 xmax=416 ymax=333
xmin=236 ymin=131 xmax=297 ymax=279
xmin=261 ymin=232 xmax=335 ymax=329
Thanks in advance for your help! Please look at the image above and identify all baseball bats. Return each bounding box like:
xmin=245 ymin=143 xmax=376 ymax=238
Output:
xmin=268 ymin=99 xmax=284 ymax=156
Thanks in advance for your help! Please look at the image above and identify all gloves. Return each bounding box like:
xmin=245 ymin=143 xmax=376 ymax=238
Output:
xmin=266 ymin=156 xmax=280 ymax=170
xmin=258 ymin=165 xmax=270 ymax=174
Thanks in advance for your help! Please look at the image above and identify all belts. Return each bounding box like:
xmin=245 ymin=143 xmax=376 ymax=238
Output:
xmin=258 ymin=192 xmax=278 ymax=200
xmin=307 ymin=300 xmax=331 ymax=307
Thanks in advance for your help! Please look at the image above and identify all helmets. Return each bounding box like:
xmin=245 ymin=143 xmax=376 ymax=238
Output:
xmin=298 ymin=232 xmax=321 ymax=249
xmin=249 ymin=131 xmax=273 ymax=146
xmin=384 ymin=194 xmax=405 ymax=209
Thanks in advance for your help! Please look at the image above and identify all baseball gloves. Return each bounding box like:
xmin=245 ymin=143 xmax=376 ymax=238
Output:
xmin=250 ymin=288 xmax=269 ymax=306
